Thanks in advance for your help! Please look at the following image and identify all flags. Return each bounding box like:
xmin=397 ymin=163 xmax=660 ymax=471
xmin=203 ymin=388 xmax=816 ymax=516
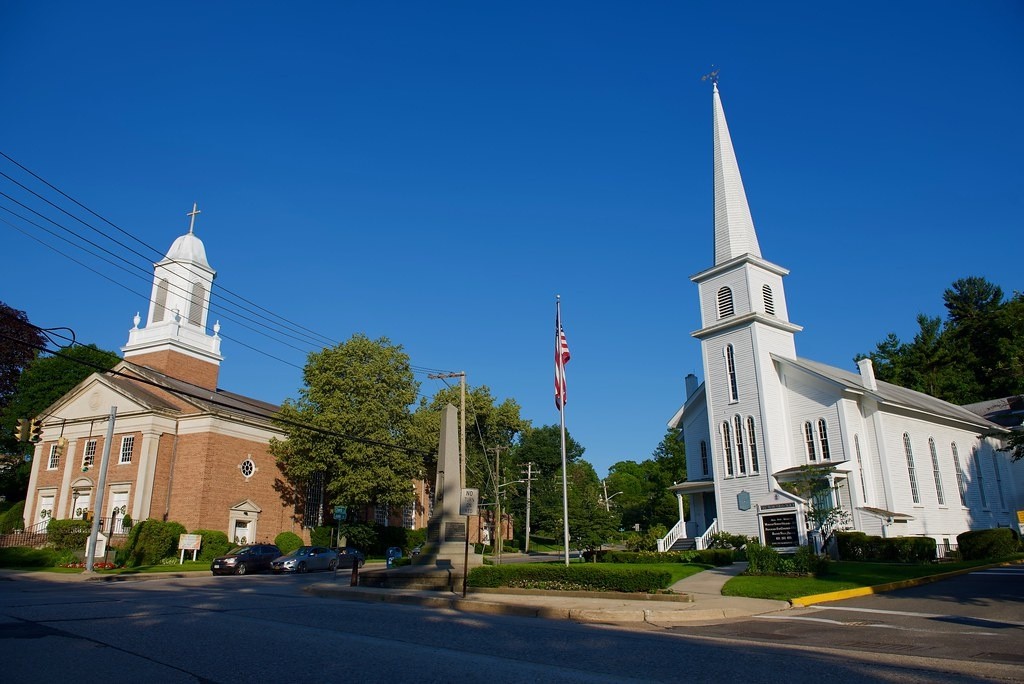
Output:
xmin=555 ymin=319 xmax=571 ymax=410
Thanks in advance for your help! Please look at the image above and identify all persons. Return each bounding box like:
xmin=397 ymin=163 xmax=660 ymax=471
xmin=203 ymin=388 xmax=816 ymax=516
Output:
xmin=341 ymin=550 xmax=346 ymax=554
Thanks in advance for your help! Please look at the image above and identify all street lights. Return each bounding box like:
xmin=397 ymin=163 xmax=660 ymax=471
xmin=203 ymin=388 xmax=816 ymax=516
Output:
xmin=599 ymin=480 xmax=623 ymax=512
xmin=487 ymin=445 xmax=525 ymax=565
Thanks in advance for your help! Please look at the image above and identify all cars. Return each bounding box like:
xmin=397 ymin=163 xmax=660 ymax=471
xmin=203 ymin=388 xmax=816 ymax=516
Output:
xmin=210 ymin=543 xmax=367 ymax=577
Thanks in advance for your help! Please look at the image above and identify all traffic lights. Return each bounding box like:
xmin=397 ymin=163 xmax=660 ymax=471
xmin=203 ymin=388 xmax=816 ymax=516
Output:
xmin=55 ymin=437 xmax=69 ymax=458
xmin=81 ymin=454 xmax=91 ymax=472
xmin=13 ymin=416 xmax=48 ymax=444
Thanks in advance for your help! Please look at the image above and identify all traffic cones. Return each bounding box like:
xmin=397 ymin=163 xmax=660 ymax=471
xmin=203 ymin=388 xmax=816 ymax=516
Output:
xmin=350 ymin=557 xmax=358 ymax=588
